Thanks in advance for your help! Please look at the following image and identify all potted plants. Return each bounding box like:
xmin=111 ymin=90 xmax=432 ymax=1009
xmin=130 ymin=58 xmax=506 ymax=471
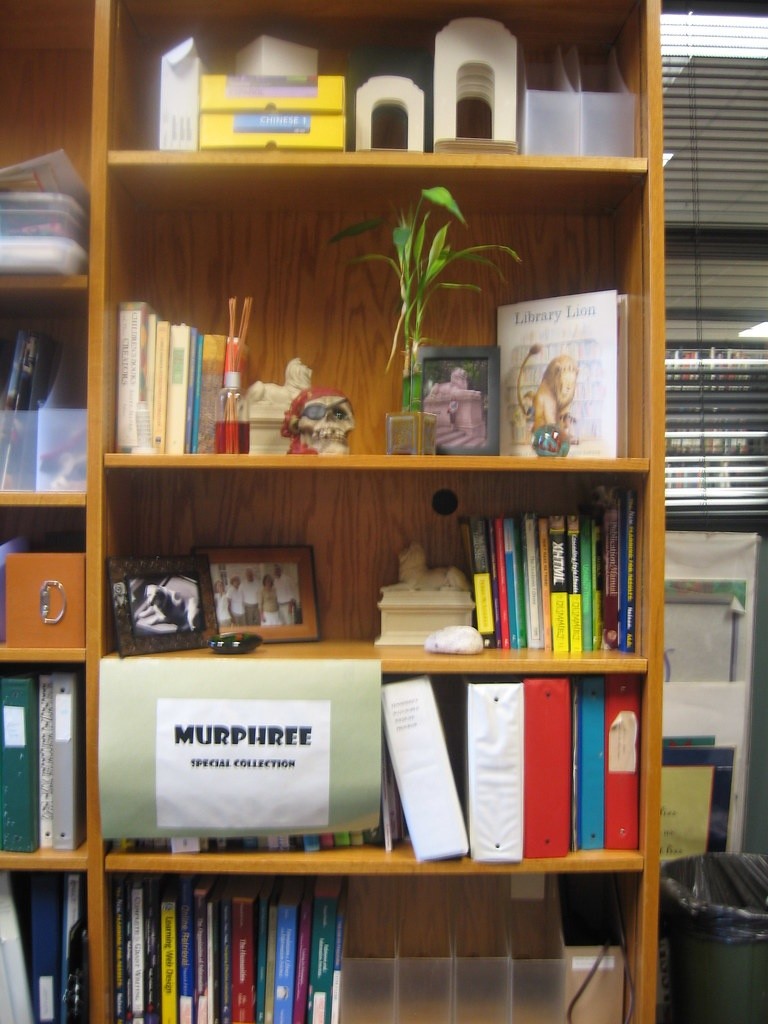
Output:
xmin=326 ymin=187 xmax=525 ymax=456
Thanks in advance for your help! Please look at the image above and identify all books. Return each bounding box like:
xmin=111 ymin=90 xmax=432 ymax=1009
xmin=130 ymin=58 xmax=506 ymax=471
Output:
xmin=0 ymin=659 xmax=382 ymax=1024
xmin=457 ymin=483 xmax=636 ymax=654
xmin=0 ymin=302 xmax=229 ymax=492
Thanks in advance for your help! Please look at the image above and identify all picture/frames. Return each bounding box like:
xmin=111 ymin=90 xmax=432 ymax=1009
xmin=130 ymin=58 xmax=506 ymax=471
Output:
xmin=106 ymin=551 xmax=220 ymax=659
xmin=194 ymin=543 xmax=320 ymax=644
xmin=411 ymin=346 xmax=501 ymax=456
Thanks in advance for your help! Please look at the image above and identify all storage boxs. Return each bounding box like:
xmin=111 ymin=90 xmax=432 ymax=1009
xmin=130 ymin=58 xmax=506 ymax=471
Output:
xmin=159 ymin=37 xmax=209 ymax=152
xmin=199 ymin=115 xmax=346 ymax=151
xmin=6 ymin=530 xmax=85 ymax=648
xmin=235 ymin=35 xmax=318 ymax=76
xmin=200 ymin=74 xmax=346 ymax=115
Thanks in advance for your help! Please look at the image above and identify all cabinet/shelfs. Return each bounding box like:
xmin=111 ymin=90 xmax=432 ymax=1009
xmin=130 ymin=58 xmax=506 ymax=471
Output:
xmin=0 ymin=0 xmax=664 ymax=1024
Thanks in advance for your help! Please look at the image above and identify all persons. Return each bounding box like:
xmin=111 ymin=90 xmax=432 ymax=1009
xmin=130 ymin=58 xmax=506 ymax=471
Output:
xmin=213 ymin=563 xmax=301 ymax=630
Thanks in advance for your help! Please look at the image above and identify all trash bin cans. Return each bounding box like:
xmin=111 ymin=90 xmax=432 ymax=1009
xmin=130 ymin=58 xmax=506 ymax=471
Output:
xmin=660 ymin=853 xmax=768 ymax=1024
